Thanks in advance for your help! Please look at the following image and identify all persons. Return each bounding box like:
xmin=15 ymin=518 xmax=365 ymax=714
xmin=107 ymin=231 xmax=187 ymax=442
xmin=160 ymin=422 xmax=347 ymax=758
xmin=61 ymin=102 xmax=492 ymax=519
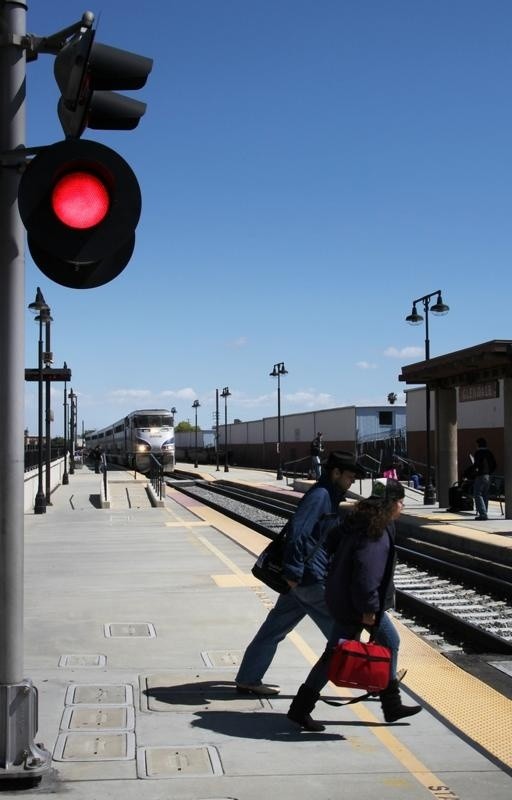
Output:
xmin=411 ymin=472 xmax=425 ymax=490
xmin=459 ymin=437 xmax=497 ymax=521
xmin=93 ymin=447 xmax=103 ymax=473
xmin=306 ymin=431 xmax=325 ymax=479
xmin=284 ymin=478 xmax=422 ymax=731
xmin=235 ymin=450 xmax=409 ymax=699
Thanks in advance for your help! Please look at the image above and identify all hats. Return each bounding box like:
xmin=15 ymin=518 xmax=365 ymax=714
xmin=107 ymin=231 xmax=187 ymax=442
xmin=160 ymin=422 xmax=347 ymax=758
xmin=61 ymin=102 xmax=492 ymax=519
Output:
xmin=318 ymin=450 xmax=364 ymax=473
xmin=372 ymin=478 xmax=404 ymax=499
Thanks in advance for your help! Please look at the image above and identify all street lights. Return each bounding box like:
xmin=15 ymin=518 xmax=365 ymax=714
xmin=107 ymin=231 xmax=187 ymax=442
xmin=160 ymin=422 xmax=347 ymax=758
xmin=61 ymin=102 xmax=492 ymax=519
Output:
xmin=192 ymin=399 xmax=201 ymax=468
xmin=406 ymin=291 xmax=449 ymax=504
xmin=27 ymin=285 xmax=76 ymax=515
xmin=268 ymin=360 xmax=289 ymax=480
xmin=170 ymin=406 xmax=177 ymax=418
xmin=218 ymin=386 xmax=230 ymax=472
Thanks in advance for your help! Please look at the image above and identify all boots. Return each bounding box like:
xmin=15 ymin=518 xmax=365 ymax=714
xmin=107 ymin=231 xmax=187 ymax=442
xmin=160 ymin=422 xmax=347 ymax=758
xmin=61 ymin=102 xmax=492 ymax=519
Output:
xmin=380 ymin=679 xmax=422 ymax=722
xmin=287 ymin=683 xmax=325 ymax=731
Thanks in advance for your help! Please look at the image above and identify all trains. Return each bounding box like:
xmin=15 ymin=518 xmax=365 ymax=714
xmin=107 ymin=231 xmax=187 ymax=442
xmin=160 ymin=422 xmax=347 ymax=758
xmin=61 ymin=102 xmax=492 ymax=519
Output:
xmin=85 ymin=408 xmax=177 ymax=475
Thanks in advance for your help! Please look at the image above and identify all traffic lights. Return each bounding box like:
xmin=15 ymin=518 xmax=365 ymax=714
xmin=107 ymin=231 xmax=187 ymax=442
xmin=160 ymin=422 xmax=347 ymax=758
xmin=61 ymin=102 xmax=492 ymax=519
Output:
xmin=15 ymin=146 xmax=142 ymax=284
xmin=89 ymin=42 xmax=150 ymax=138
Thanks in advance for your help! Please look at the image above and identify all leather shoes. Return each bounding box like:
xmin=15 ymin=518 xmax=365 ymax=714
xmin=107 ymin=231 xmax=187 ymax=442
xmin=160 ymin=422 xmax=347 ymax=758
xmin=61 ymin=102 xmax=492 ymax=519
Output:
xmin=236 ymin=682 xmax=280 ymax=695
xmin=396 ymin=668 xmax=408 ymax=684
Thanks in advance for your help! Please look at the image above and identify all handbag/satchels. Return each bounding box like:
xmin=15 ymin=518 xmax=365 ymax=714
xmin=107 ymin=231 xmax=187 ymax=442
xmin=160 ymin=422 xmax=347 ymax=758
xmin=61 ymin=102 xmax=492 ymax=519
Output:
xmin=252 ymin=537 xmax=290 ymax=595
xmin=328 ymin=639 xmax=392 ymax=692
